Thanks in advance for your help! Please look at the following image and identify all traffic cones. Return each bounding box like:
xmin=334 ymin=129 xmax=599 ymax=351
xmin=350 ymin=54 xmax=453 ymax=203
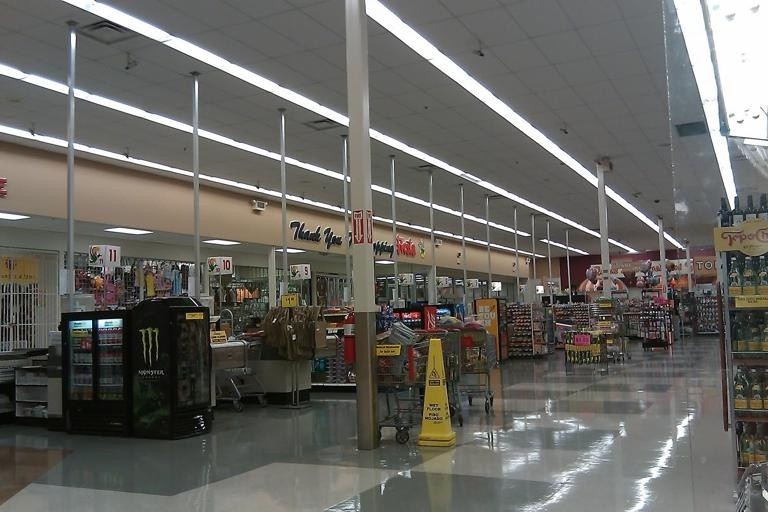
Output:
xmin=417 ymin=337 xmax=456 ymax=448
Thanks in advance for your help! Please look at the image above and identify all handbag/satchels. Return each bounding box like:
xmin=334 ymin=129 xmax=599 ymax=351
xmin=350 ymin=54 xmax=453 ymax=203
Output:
xmin=261 ymin=306 xmax=329 ymax=362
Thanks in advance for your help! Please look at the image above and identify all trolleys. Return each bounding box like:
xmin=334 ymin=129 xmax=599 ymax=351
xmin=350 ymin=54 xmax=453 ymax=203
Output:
xmin=376 ymin=326 xmax=497 ymax=443
xmin=214 ymin=329 xmax=268 ymax=413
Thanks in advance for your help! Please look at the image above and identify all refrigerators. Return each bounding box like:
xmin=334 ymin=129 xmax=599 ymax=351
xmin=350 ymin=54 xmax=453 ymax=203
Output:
xmin=59 ymin=297 xmax=212 ymax=438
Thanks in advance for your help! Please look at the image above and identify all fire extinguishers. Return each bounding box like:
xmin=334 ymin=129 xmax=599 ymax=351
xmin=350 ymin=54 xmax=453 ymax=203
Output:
xmin=344 ymin=306 xmax=355 ymax=363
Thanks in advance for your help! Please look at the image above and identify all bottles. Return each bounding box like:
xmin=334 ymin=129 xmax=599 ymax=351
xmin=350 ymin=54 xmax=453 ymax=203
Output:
xmin=716 ymin=193 xmax=767 ymax=468
xmin=71 ymin=327 xmax=123 ymax=401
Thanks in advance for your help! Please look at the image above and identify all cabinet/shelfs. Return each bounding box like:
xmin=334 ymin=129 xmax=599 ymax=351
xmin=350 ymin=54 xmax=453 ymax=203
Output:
xmin=310 ymin=301 xmax=510 ymax=388
xmin=506 ymin=300 xmax=672 ymax=377
xmin=713 ymin=211 xmax=767 ymax=474
